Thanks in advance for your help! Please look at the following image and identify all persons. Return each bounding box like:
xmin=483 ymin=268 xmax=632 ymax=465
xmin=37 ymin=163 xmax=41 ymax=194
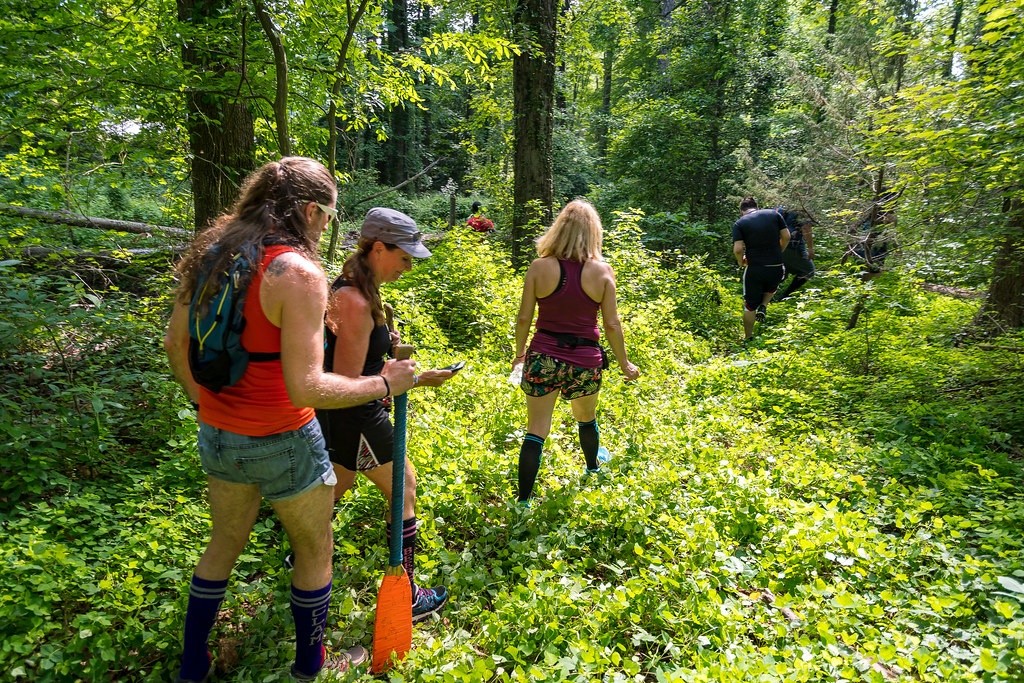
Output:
xmin=733 ymin=197 xmax=815 ymax=341
xmin=284 ymin=206 xmax=459 ymax=623
xmin=511 ymin=200 xmax=640 ymax=510
xmin=845 ymin=188 xmax=897 ymax=274
xmin=465 ymin=201 xmax=493 ymax=233
xmin=164 ymin=158 xmax=416 ymax=682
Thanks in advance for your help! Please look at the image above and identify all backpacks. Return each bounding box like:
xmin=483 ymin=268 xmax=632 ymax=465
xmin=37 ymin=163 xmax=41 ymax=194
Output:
xmin=467 ymin=215 xmax=481 ymax=231
xmin=188 ymin=233 xmax=286 ymax=391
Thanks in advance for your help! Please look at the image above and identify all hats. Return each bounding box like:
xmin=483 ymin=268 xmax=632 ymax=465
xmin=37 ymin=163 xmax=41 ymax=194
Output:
xmin=360 ymin=206 xmax=434 ymax=259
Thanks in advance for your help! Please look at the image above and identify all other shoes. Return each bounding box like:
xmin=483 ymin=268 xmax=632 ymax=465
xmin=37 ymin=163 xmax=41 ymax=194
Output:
xmin=756 ymin=305 xmax=767 ymax=321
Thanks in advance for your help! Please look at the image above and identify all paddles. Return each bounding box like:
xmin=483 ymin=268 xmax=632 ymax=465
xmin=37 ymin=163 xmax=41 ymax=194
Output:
xmin=372 ymin=342 xmax=418 ymax=675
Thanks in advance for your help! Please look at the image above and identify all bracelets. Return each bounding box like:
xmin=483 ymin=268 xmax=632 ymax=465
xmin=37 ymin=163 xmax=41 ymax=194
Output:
xmin=379 ymin=374 xmax=392 ymax=405
xmin=413 ymin=375 xmax=418 ymax=383
xmin=516 ymin=354 xmax=525 ymax=359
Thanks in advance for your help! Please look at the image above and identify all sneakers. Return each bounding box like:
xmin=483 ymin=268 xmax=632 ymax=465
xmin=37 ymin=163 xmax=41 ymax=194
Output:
xmin=515 ymin=501 xmax=530 ymax=510
xmin=208 ymin=652 xmax=225 ymax=679
xmin=586 ymin=446 xmax=609 ymax=474
xmin=290 ymin=644 xmax=370 ymax=683
xmin=412 ymin=582 xmax=448 ymax=622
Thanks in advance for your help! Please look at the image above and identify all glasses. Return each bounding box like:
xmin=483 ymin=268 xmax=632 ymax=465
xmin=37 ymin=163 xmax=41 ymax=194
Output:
xmin=392 ymin=231 xmax=423 ymax=243
xmin=295 ymin=199 xmax=340 ymax=223
